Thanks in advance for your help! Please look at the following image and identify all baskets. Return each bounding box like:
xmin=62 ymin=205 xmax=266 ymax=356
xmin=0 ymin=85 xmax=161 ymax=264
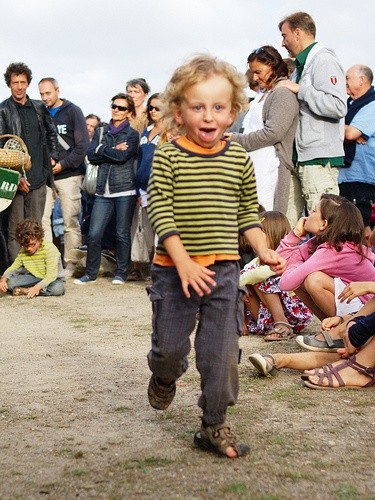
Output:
xmin=0 ymin=134 xmax=32 ymax=172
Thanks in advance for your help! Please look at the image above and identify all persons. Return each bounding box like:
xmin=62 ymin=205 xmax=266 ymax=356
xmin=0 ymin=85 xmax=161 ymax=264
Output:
xmin=334 ymin=62 xmax=375 ymax=235
xmin=0 ymin=217 xmax=66 ymax=297
xmin=277 ymin=55 xmax=300 ymax=83
xmin=35 ymin=78 xmax=151 ymax=286
xmin=125 ymin=92 xmax=176 ymax=286
xmin=0 ymin=63 xmax=58 ymax=282
xmin=192 ymin=192 xmax=374 ymax=395
xmin=146 ymin=54 xmax=288 ymax=458
xmin=220 ymin=45 xmax=306 ymax=239
xmin=272 ymin=11 xmax=350 ymax=243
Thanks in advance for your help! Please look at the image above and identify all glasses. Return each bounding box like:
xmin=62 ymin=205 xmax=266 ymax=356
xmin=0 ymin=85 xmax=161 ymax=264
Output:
xmin=23 ymin=237 xmax=38 ymax=249
xmin=112 ymin=103 xmax=130 ymax=111
xmin=255 ymin=47 xmax=271 ymax=58
xmin=148 ymin=104 xmax=162 ymax=111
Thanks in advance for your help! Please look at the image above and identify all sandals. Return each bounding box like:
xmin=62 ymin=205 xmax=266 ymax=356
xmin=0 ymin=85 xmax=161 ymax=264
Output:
xmin=148 ymin=373 xmax=176 ymax=410
xmin=264 ymin=321 xmax=295 ymax=341
xmin=300 ymin=354 xmax=375 ymax=389
xmin=12 ymin=287 xmax=26 ymax=296
xmin=194 ymin=416 xmax=250 ymax=458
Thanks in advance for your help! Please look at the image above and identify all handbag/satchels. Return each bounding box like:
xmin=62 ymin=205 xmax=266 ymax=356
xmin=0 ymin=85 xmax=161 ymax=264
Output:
xmin=82 ymin=126 xmax=104 ymax=194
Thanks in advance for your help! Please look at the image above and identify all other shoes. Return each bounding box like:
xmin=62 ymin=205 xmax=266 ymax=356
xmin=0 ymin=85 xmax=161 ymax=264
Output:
xmin=112 ymin=275 xmax=125 ymax=285
xmin=145 ymin=276 xmax=153 ymax=285
xmin=126 ymin=269 xmax=142 ymax=280
xmin=296 ymin=331 xmax=346 ymax=351
xmin=249 ymin=353 xmax=280 ymax=377
xmin=73 ymin=275 xmax=96 ymax=284
